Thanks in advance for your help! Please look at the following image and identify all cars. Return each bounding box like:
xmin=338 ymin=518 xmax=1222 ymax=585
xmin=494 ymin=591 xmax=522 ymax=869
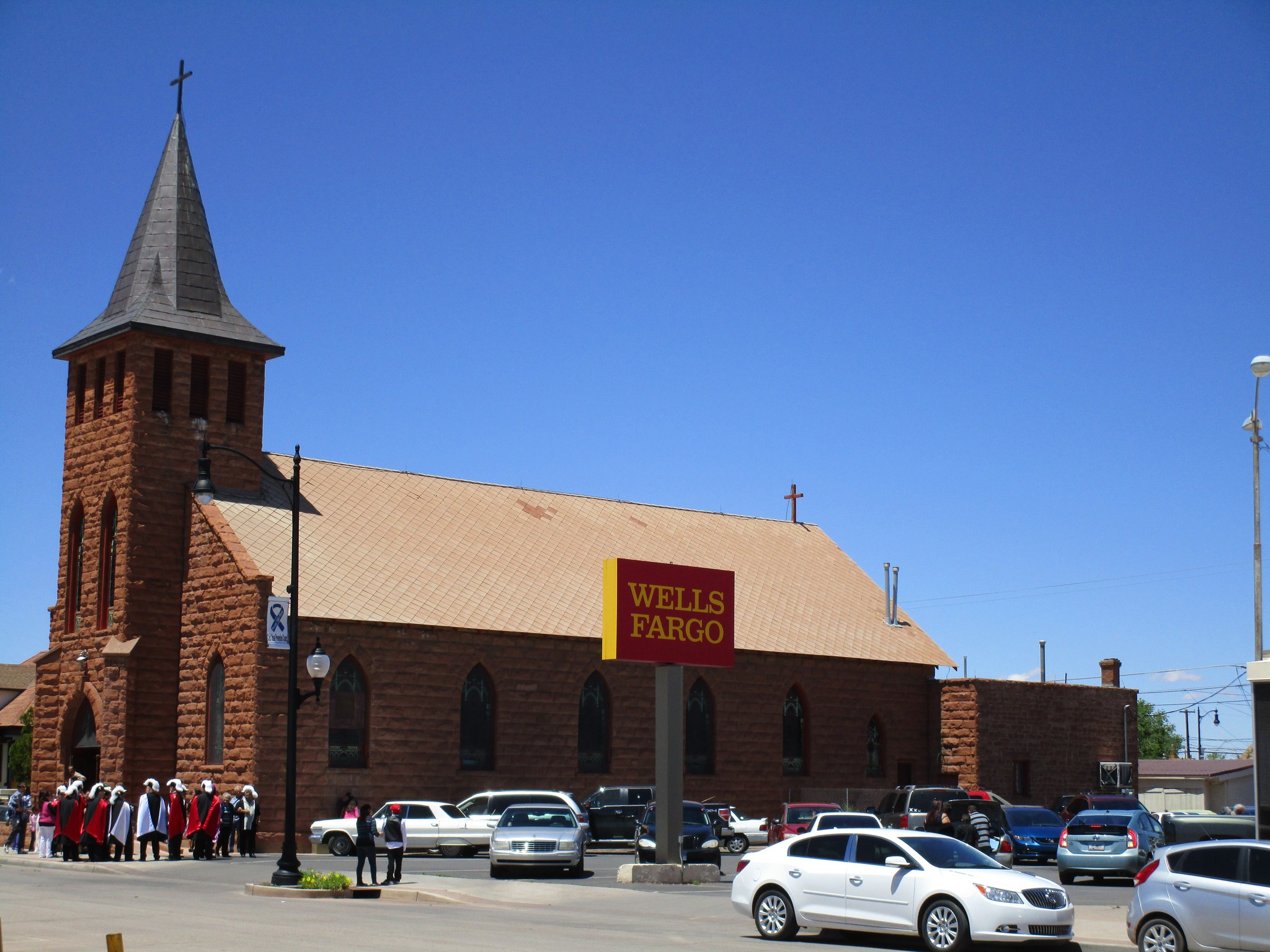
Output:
xmin=730 ymin=827 xmax=1075 ymax=952
xmin=1127 ymin=839 xmax=1270 ymax=952
xmin=1051 ymin=791 xmax=1254 ymax=824
xmin=797 ymin=812 xmax=884 ymax=833
xmin=768 ymin=802 xmax=843 ymax=847
xmin=967 ymin=790 xmax=1070 ymax=862
xmin=1057 ymin=809 xmax=1165 ymax=885
xmin=487 ymin=803 xmax=589 ymax=878
xmin=948 ymin=799 xmax=1013 ymax=870
xmin=719 ymin=806 xmax=769 ymax=853
xmin=308 ymin=801 xmax=502 ymax=858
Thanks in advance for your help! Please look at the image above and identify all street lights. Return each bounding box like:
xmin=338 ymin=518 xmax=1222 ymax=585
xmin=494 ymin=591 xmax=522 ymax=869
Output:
xmin=1197 ymin=706 xmax=1220 ymax=760
xmin=191 ymin=431 xmax=330 ymax=886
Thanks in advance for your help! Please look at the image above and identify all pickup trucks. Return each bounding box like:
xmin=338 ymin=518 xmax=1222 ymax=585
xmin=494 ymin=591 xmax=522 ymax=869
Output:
xmin=581 ymin=786 xmax=734 ymax=853
xmin=1161 ymin=814 xmax=1256 ymax=846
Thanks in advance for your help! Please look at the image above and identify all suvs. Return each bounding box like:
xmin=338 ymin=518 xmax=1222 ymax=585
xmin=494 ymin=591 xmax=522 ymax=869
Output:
xmin=425 ymin=790 xmax=592 ymax=854
xmin=866 ymin=785 xmax=971 ymax=831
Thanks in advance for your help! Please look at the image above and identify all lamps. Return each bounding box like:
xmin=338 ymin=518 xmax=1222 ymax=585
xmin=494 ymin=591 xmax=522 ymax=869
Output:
xmin=77 ymin=650 xmax=88 ymax=661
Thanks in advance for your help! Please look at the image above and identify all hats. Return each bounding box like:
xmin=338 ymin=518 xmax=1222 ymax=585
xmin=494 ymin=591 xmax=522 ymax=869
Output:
xmin=143 ymin=778 xmax=160 ymax=792
xmin=201 ymin=779 xmax=216 ymax=794
xmin=390 ymin=804 xmax=401 ymax=814
xmin=166 ymin=778 xmax=183 ymax=791
xmin=56 ymin=780 xmax=127 ymax=805
xmin=181 ymin=785 xmax=189 ymax=792
xmin=345 ymin=793 xmax=353 ymax=798
xmin=241 ymin=785 xmax=258 ymax=798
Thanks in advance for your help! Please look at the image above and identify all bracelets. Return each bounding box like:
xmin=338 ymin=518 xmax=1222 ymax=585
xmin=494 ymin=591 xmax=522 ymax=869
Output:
xmin=258 ymin=821 xmax=261 ymax=822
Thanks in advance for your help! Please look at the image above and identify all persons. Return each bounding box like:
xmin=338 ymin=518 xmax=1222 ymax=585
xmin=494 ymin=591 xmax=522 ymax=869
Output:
xmin=924 ymin=799 xmax=993 ymax=858
xmin=356 ymin=804 xmax=384 ymax=887
xmin=1220 ymin=803 xmax=1245 ymax=815
xmin=336 ymin=792 xmax=360 ymax=819
xmin=3 ymin=765 xmax=261 ymax=862
xmin=381 ymin=804 xmax=407 ymax=885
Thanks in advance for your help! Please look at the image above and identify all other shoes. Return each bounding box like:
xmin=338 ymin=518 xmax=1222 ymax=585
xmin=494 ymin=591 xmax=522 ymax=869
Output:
xmin=15 ymin=848 xmax=18 ymax=852
xmin=222 ymin=855 xmax=231 ymax=857
xmin=249 ymin=853 xmax=256 ymax=857
xmin=229 ymin=849 xmax=234 ymax=852
xmin=241 ymin=854 xmax=245 ymax=857
xmin=394 ymin=879 xmax=400 ymax=884
xmin=381 ymin=879 xmax=393 ymax=885
xmin=356 ymin=883 xmax=367 ymax=886
xmin=372 ymin=883 xmax=380 ymax=886
xmin=12 ymin=848 xmax=14 ymax=851
xmin=4 ymin=844 xmax=10 ymax=853
xmin=18 ymin=848 xmax=29 ymax=854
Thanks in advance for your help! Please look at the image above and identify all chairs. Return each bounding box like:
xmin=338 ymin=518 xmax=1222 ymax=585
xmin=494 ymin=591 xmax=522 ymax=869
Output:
xmin=551 ymin=816 xmax=566 ymax=824
xmin=790 ymin=809 xmax=800 ymax=822
xmin=514 ymin=813 xmax=528 ymax=822
